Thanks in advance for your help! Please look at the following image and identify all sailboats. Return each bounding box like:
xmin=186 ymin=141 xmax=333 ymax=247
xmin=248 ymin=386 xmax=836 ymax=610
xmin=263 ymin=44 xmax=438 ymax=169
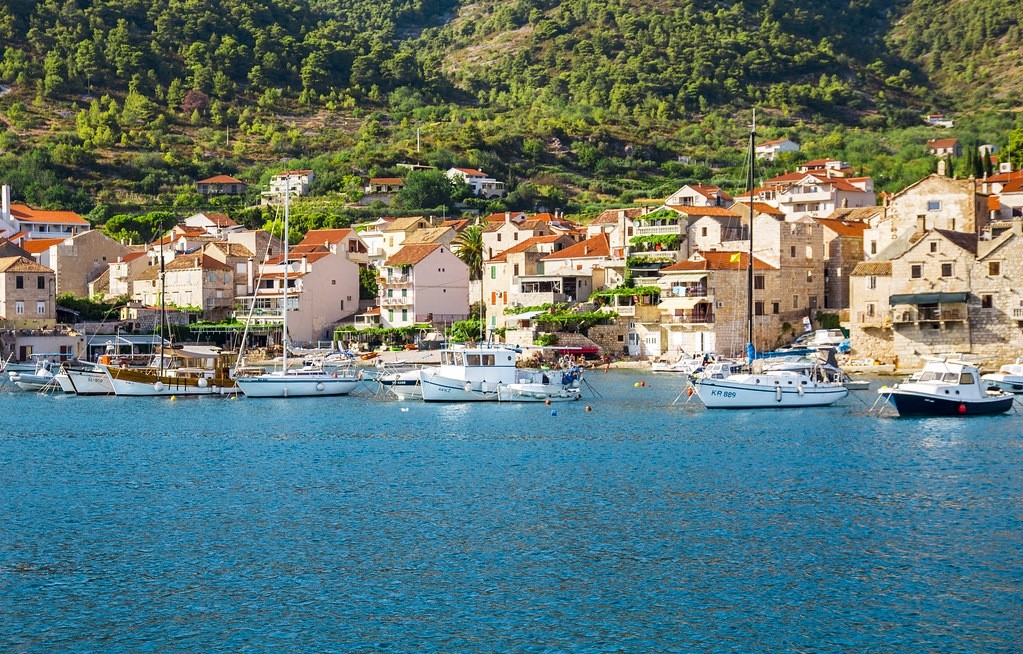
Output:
xmin=686 ymin=107 xmax=850 ymax=411
xmin=98 ymin=221 xmax=270 ymax=398
xmin=229 ymin=174 xmax=363 ymax=398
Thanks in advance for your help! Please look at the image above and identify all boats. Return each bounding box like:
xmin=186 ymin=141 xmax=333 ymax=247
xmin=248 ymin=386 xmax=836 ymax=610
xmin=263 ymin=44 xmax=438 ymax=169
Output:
xmin=301 ymin=341 xmax=355 ymax=367
xmin=981 ymin=356 xmax=1023 ymax=395
xmin=877 ymin=351 xmax=1015 ymax=419
xmin=54 ymin=341 xmax=128 ymax=396
xmin=419 ymin=325 xmax=584 ymax=404
xmin=2 ymin=351 xmax=97 ymax=391
xmin=651 ymin=315 xmax=871 ymax=393
xmin=376 ymin=368 xmax=423 ymax=401
